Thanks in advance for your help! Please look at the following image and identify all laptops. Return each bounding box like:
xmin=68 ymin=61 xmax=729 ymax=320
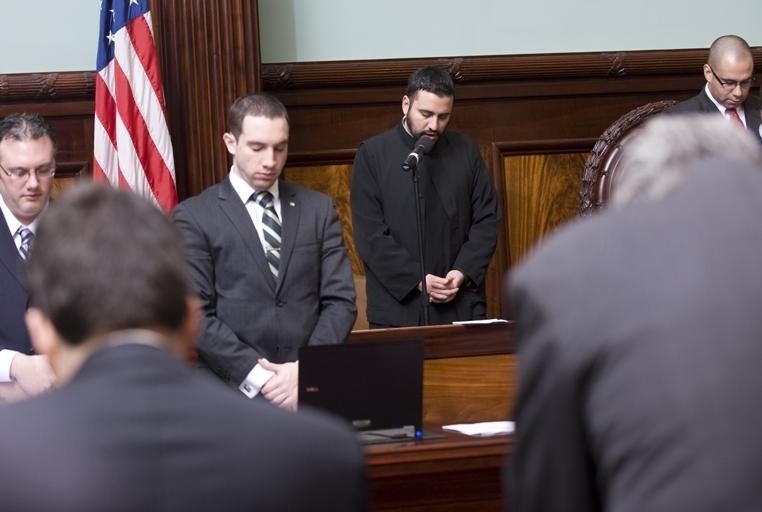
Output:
xmin=297 ymin=339 xmax=425 ymax=441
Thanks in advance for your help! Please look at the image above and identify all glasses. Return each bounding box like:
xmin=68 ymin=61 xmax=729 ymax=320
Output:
xmin=0 ymin=164 xmax=57 ymax=180
xmin=710 ymin=66 xmax=755 ymax=88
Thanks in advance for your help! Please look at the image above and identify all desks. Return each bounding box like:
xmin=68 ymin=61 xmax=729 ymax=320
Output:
xmin=347 ymin=319 xmax=522 ymax=423
xmin=351 ymin=420 xmax=515 ymax=510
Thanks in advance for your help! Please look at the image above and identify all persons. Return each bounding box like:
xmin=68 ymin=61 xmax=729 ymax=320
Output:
xmin=169 ymin=93 xmax=358 ymax=414
xmin=500 ymin=112 xmax=762 ymax=512
xmin=350 ymin=64 xmax=502 ymax=330
xmin=1 ymin=181 xmax=378 ymax=511
xmin=660 ymin=34 xmax=762 ymax=148
xmin=0 ymin=111 xmax=58 ymax=404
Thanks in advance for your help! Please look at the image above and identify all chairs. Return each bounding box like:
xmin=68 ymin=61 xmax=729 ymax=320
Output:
xmin=576 ymin=98 xmax=682 ymax=218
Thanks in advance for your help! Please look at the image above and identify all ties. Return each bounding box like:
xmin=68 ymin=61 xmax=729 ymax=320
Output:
xmin=250 ymin=190 xmax=283 ymax=284
xmin=727 ymin=107 xmax=744 ymax=130
xmin=17 ymin=226 xmax=36 ymax=260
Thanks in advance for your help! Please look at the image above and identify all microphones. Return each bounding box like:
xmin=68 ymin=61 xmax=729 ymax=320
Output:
xmin=400 ymin=137 xmax=433 ymax=171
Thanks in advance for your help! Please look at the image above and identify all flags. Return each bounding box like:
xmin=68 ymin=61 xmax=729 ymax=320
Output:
xmin=92 ymin=0 xmax=178 ymax=221
